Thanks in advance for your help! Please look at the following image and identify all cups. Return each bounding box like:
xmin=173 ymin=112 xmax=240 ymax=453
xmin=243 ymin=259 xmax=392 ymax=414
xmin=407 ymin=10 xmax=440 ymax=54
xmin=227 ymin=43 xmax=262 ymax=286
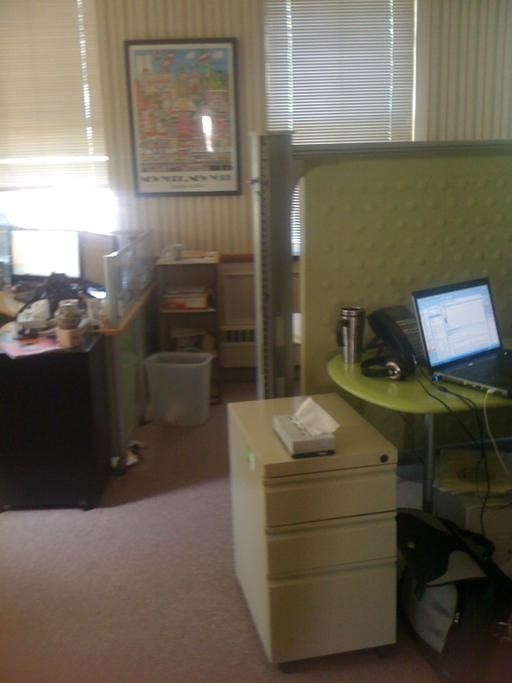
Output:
xmin=162 ymin=244 xmax=183 ymax=260
xmin=337 ymin=307 xmax=364 ymax=365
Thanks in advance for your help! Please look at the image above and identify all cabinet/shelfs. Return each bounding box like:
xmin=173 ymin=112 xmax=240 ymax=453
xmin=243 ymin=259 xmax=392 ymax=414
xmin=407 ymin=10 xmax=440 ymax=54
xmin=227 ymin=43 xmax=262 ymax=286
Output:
xmin=1 ymin=331 xmax=108 ymax=511
xmin=154 ymin=250 xmax=224 ymax=406
xmin=226 ymin=390 xmax=399 ymax=675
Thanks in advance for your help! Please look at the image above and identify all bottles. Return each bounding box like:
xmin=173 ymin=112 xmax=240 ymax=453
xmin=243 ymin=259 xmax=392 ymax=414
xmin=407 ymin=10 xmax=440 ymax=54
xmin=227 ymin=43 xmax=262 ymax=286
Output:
xmin=55 ymin=299 xmax=84 ymax=349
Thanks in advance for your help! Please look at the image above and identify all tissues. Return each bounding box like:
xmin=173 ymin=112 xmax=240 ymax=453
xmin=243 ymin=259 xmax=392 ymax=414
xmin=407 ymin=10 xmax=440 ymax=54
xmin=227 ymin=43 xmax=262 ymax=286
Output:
xmin=272 ymin=395 xmax=341 ymax=458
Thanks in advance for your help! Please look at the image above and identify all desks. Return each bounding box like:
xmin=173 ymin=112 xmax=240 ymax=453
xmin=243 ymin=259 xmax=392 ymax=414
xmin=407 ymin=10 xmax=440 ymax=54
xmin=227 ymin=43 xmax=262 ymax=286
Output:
xmin=1 ymin=230 xmax=157 ymax=471
xmin=326 ymin=347 xmax=510 ymax=513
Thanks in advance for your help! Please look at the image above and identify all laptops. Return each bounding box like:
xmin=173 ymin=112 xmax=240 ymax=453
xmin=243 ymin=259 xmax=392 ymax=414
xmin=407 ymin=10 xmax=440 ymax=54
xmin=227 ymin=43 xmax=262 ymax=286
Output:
xmin=409 ymin=276 xmax=510 ymax=399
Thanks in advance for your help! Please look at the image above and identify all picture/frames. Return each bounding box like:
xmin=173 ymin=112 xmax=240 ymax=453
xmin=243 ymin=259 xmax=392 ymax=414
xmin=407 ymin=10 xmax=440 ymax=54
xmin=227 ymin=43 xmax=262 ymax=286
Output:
xmin=123 ymin=37 xmax=242 ymax=198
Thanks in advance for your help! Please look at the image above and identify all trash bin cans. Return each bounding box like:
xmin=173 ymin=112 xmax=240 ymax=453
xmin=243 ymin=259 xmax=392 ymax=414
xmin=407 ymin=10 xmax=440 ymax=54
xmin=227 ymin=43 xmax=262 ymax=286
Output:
xmin=144 ymin=350 xmax=214 ymax=428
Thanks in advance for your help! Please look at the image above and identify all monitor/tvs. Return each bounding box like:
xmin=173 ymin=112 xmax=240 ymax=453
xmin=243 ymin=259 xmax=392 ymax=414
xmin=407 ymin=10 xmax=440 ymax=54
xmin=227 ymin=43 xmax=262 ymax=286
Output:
xmin=79 ymin=231 xmax=117 ymax=288
xmin=10 ymin=229 xmax=81 ymax=283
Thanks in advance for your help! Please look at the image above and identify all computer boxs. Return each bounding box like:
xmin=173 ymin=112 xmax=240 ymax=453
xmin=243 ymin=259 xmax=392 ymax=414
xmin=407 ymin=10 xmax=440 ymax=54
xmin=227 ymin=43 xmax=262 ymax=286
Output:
xmin=431 ymin=484 xmax=512 ymax=578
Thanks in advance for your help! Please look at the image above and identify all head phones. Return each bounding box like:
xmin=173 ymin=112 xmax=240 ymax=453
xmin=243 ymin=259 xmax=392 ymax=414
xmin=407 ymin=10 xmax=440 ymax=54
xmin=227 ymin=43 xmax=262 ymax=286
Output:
xmin=360 ymin=354 xmax=415 ymax=381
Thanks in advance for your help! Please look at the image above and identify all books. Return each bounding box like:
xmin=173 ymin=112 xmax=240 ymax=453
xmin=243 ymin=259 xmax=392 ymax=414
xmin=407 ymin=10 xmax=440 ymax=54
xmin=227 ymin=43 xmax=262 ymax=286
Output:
xmin=160 ymin=281 xmax=212 ymax=299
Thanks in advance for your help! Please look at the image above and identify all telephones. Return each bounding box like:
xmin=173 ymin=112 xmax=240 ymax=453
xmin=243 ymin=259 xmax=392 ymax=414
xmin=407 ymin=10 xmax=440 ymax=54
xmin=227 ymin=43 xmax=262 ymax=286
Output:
xmin=367 ymin=304 xmax=427 ymax=377
